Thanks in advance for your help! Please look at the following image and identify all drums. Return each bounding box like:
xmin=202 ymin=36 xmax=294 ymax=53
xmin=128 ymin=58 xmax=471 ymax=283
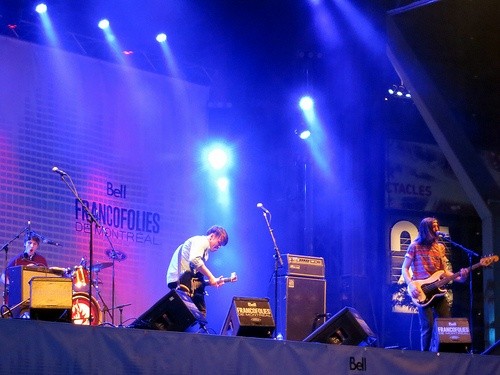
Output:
xmin=71 ymin=292 xmax=102 ymax=327
xmin=64 ymin=265 xmax=90 ymax=291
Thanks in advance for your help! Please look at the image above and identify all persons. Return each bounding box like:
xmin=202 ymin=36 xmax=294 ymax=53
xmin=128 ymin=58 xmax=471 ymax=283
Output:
xmin=402 ymin=217 xmax=469 ymax=352
xmin=1 ymin=232 xmax=49 ymax=284
xmin=167 ymin=224 xmax=229 ymax=327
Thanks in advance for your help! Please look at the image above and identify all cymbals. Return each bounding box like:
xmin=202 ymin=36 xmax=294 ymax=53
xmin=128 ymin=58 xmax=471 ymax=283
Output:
xmin=90 ymin=262 xmax=114 ymax=271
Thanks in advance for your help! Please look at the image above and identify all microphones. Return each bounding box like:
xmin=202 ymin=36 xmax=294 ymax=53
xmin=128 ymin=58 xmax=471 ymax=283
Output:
xmin=257 ymin=202 xmax=270 ymax=214
xmin=43 ymin=240 xmax=60 ymax=246
xmin=28 ymin=220 xmax=31 ymax=240
xmin=436 ymin=231 xmax=445 ymax=237
xmin=52 ymin=167 xmax=69 ymax=176
xmin=80 ymin=257 xmax=84 ymax=264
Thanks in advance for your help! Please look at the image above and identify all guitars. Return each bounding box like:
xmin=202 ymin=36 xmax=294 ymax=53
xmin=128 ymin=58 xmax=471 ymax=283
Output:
xmin=168 ymin=271 xmax=237 ymax=301
xmin=407 ymin=253 xmax=499 ymax=307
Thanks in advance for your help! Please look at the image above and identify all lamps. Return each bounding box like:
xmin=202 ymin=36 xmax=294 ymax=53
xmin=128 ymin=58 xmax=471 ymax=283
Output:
xmin=384 ymin=84 xmax=411 ymax=100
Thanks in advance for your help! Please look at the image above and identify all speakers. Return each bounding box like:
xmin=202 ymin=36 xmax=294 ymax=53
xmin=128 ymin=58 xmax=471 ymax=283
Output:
xmin=127 ymin=289 xmax=203 ymax=332
xmin=29 ymin=277 xmax=73 ymax=321
xmin=429 ymin=317 xmax=470 ymax=352
xmin=303 ymin=306 xmax=375 ymax=345
xmin=273 ymin=275 xmax=326 ymax=342
xmin=220 ymin=297 xmax=276 ymax=335
